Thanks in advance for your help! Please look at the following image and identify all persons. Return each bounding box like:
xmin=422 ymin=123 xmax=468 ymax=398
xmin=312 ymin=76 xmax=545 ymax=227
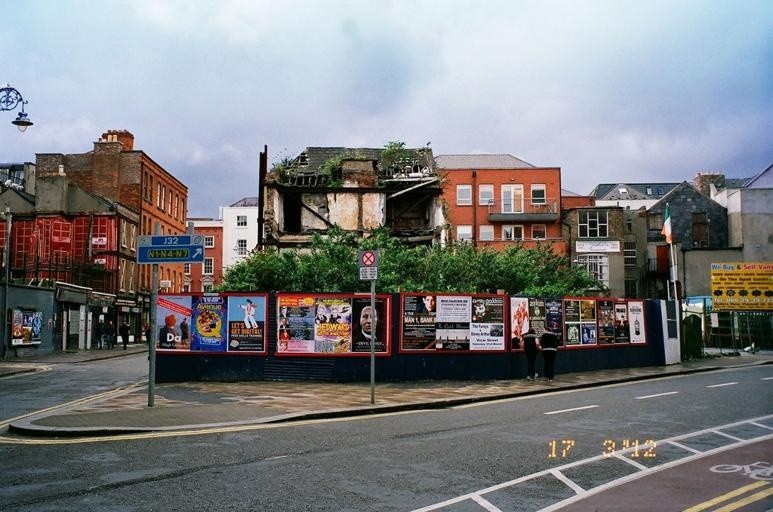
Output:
xmin=520 ymin=328 xmax=542 ymax=384
xmin=278 ymin=340 xmax=288 ymax=352
xmin=414 ymin=295 xmax=435 ymax=313
xmin=104 ymin=319 xmax=116 ymax=349
xmin=278 ymin=317 xmax=288 ymax=328
xmin=314 ymin=304 xmax=327 ymax=325
xmin=144 ymin=323 xmax=150 ymax=347
xmin=538 ymin=327 xmax=559 ymax=381
xmin=118 ymin=318 xmax=130 ymax=350
xmin=279 ymin=329 xmax=288 ymax=339
xmin=94 ymin=319 xmax=106 ymax=350
xmin=328 ymin=306 xmax=342 ymax=325
xmin=352 ymin=306 xmax=384 ymax=352
xmin=179 ymin=317 xmax=188 ymax=345
xmin=279 ymin=307 xmax=288 ymax=317
xmin=159 ymin=314 xmax=177 ymax=349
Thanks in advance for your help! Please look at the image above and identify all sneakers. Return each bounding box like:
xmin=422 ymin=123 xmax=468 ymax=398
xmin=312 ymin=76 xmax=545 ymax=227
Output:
xmin=526 ymin=373 xmax=538 ymax=381
xmin=547 ymin=378 xmax=553 ymax=382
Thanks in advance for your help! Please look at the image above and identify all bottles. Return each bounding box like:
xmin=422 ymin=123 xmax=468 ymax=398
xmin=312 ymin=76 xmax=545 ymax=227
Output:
xmin=23 ymin=316 xmax=28 ymax=326
xmin=583 ymin=329 xmax=589 ymax=343
xmin=634 ymin=316 xmax=640 ymax=335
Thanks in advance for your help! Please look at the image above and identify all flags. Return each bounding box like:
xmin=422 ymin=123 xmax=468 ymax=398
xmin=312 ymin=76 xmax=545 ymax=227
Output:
xmin=660 ymin=204 xmax=673 ymax=244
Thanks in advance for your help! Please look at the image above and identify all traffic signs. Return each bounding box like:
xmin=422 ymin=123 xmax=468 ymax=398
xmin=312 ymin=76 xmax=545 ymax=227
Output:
xmin=136 ymin=236 xmax=205 ymax=262
